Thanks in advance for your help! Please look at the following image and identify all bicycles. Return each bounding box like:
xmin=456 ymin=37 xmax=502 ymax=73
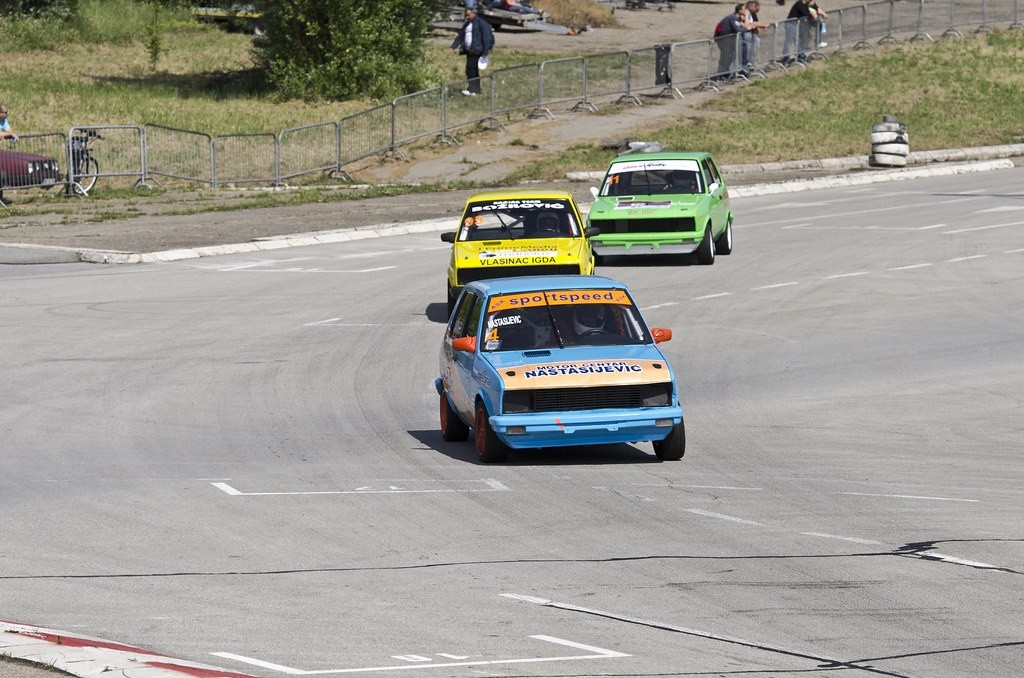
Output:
xmin=64 ymin=127 xmax=105 ymax=195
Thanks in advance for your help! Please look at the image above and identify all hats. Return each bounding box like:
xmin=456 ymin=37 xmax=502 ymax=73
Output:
xmin=477 ymin=55 xmax=489 ymax=70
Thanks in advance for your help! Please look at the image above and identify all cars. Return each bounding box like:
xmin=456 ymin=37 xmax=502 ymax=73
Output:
xmin=439 ymin=189 xmax=600 ymax=322
xmin=586 ymin=152 xmax=736 ymax=266
xmin=434 ymin=275 xmax=686 ymax=461
xmin=0 ymin=147 xmax=59 ymax=187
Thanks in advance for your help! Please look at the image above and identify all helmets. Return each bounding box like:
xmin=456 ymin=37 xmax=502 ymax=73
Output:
xmin=537 ymin=213 xmax=559 ymax=233
xmin=572 ymin=303 xmax=607 ymax=336
xmin=672 ymin=170 xmax=694 ymax=188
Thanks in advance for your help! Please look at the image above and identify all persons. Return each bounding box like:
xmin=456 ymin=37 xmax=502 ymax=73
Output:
xmin=536 ymin=212 xmax=562 ymax=233
xmin=0 ymin=104 xmax=19 ymax=205
xmin=714 ymin=0 xmax=770 ymax=81
xmin=464 ymin=0 xmax=545 ymax=22
xmin=447 ymin=8 xmax=489 ymax=96
xmin=662 ymin=170 xmax=692 ymax=192
xmin=572 ymin=304 xmax=606 ymax=336
xmin=782 ymin=0 xmax=829 ymax=67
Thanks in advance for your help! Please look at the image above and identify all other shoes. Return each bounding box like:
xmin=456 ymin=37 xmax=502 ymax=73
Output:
xmin=462 ymin=89 xmax=477 ymax=96
xmin=818 ymin=41 xmax=828 ymax=48
xmin=799 ymin=54 xmax=808 ymax=60
